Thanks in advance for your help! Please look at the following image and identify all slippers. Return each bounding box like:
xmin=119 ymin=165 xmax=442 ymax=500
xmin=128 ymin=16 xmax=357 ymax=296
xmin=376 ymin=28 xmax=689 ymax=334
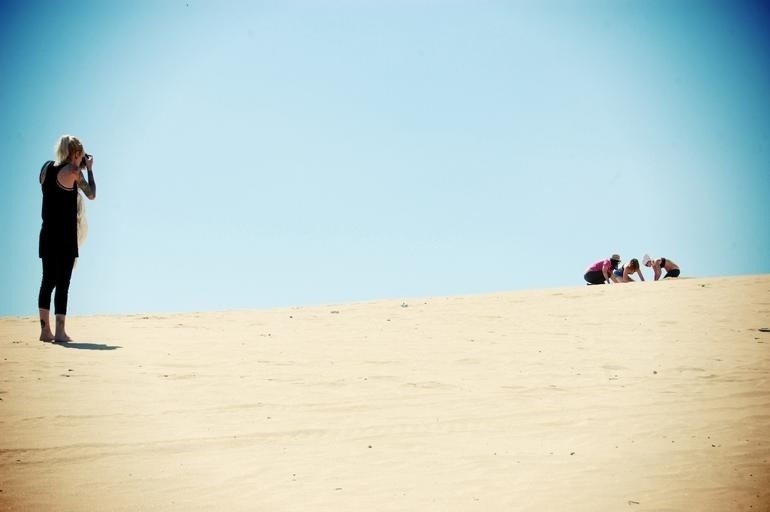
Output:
xmin=40 ymin=333 xmax=70 ymax=342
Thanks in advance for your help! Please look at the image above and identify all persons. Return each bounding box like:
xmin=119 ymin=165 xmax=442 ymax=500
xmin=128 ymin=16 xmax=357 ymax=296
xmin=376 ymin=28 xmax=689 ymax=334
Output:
xmin=613 ymin=257 xmax=645 ymax=284
xmin=641 ymin=254 xmax=680 ymax=281
xmin=38 ymin=133 xmax=96 ymax=345
xmin=584 ymin=254 xmax=622 ymax=284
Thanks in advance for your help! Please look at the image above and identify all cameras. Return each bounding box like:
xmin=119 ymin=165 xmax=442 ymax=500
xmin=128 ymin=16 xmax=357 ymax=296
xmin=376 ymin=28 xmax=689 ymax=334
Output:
xmin=79 ymin=154 xmax=89 ymax=166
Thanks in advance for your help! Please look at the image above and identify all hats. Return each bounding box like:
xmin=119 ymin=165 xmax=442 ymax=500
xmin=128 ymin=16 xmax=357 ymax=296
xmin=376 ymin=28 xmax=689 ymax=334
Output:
xmin=610 ymin=254 xmax=621 ymax=261
xmin=641 ymin=254 xmax=651 ymax=265
